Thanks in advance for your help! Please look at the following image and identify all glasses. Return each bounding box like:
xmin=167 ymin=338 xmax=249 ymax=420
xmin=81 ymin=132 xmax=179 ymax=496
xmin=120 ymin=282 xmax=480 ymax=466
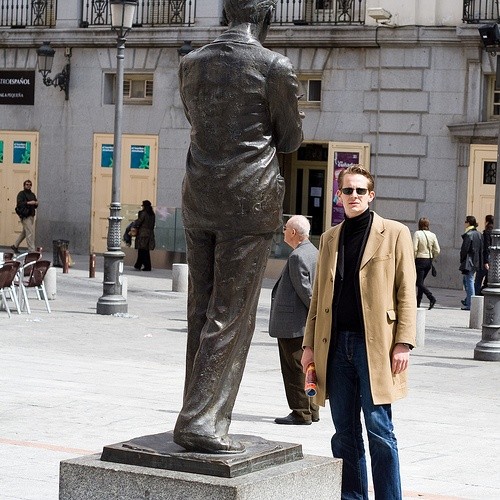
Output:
xmin=282 ymin=226 xmax=292 ymax=232
xmin=26 ymin=184 xmax=31 ymax=186
xmin=464 ymin=220 xmax=468 ymax=224
xmin=341 ymin=188 xmax=368 ymax=195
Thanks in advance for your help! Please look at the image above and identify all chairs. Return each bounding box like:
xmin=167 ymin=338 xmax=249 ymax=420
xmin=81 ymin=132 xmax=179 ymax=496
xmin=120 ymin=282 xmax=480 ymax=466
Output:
xmin=0 ymin=252 xmax=52 ymax=318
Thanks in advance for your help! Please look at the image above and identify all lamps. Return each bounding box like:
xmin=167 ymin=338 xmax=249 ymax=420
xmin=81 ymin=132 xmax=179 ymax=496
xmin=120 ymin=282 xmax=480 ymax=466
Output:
xmin=367 ymin=7 xmax=392 ymax=27
xmin=479 ymin=22 xmax=500 ymax=58
xmin=36 ymin=39 xmax=70 ymax=101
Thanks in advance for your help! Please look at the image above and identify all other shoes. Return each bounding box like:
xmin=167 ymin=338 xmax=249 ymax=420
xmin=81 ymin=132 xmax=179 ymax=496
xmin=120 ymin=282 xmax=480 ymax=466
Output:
xmin=428 ymin=299 xmax=436 ymax=310
xmin=11 ymin=245 xmax=20 ymax=253
xmin=461 ymin=306 xmax=470 ymax=310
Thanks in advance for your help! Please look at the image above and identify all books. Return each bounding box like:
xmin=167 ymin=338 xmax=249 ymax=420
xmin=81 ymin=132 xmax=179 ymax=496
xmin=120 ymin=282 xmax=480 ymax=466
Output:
xmin=304 ymin=362 xmax=317 ymax=397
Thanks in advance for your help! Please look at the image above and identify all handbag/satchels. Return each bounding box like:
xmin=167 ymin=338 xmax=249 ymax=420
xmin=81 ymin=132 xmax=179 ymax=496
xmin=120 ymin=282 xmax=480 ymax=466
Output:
xmin=431 ymin=264 xmax=436 ymax=276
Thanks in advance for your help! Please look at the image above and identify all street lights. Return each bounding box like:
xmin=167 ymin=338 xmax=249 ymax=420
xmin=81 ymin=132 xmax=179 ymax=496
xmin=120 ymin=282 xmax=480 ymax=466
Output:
xmin=94 ymin=1 xmax=137 ymax=315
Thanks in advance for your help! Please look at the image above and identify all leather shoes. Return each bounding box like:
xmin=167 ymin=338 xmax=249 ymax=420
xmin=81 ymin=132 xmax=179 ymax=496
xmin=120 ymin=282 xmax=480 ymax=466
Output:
xmin=275 ymin=412 xmax=312 ymax=424
xmin=313 ymin=418 xmax=319 ymax=422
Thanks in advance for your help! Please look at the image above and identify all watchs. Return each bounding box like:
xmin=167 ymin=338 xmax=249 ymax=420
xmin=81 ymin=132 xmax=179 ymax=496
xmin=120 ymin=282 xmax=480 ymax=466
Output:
xmin=403 ymin=343 xmax=409 ymax=347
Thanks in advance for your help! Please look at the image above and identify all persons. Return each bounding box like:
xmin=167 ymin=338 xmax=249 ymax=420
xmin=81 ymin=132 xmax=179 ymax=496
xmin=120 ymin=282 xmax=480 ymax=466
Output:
xmin=12 ymin=180 xmax=39 ymax=254
xmin=300 ymin=165 xmax=416 ymax=500
xmin=459 ymin=216 xmax=483 ymax=310
xmin=474 ymin=214 xmax=494 ymax=296
xmin=130 ymin=200 xmax=155 ymax=271
xmin=174 ymin=0 xmax=305 ymax=455
xmin=412 ymin=217 xmax=440 ymax=310
xmin=269 ymin=215 xmax=319 ymax=425
